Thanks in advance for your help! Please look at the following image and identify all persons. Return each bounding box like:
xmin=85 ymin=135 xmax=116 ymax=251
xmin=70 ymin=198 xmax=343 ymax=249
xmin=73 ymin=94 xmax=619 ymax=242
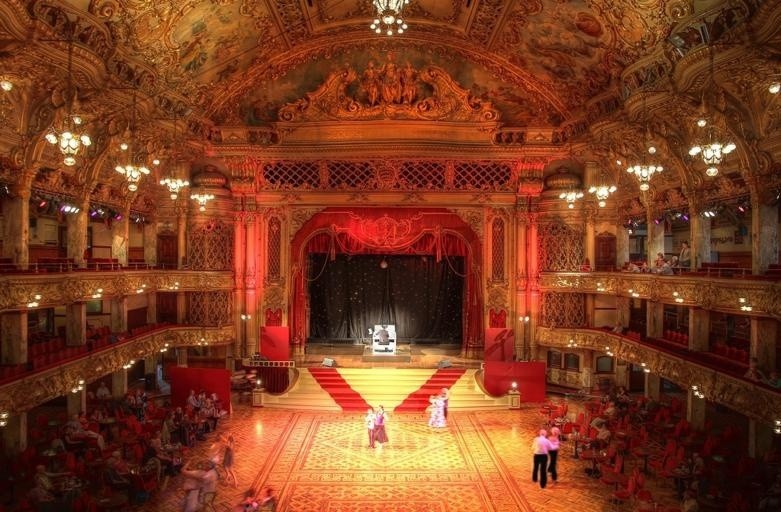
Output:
xmin=365 ymin=406 xmax=376 ymax=449
xmin=376 ymin=325 xmax=389 ymax=345
xmin=598 ymin=240 xmax=693 ymax=276
xmin=428 ymin=394 xmax=445 ymax=428
xmin=362 ymin=50 xmax=419 ymax=107
xmin=441 ymin=387 xmax=449 ymax=420
xmin=547 ymin=427 xmax=561 ymax=483
xmin=531 ymin=429 xmax=554 ymax=488
xmin=375 ymin=406 xmax=388 ymax=444
xmin=590 ymin=357 xmax=780 ymax=511
xmin=33 ymin=380 xmax=277 ymax=512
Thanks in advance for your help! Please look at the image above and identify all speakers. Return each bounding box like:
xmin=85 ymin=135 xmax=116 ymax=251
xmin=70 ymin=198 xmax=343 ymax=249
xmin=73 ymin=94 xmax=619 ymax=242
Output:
xmin=437 ymin=360 xmax=452 ymax=368
xmin=322 ymin=358 xmax=337 ymax=367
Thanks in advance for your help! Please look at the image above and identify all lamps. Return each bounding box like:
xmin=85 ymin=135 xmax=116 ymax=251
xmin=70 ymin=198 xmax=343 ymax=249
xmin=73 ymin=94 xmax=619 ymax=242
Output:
xmin=558 ymin=21 xmax=738 ymax=210
xmin=44 ymin=35 xmax=217 ymax=214
xmin=368 ymin=0 xmax=410 ymax=36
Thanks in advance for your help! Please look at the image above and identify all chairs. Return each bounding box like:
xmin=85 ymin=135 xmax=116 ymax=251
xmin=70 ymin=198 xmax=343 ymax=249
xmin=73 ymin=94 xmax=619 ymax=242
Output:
xmin=0 ymin=389 xmax=194 ymax=511
xmin=0 ymin=321 xmax=173 ymax=387
xmin=633 ymin=260 xmax=781 ymax=282
xmin=625 ymin=330 xmax=750 ymax=377
xmin=540 ymin=394 xmax=781 ymax=512
xmin=0 ymin=257 xmax=145 ymax=275
